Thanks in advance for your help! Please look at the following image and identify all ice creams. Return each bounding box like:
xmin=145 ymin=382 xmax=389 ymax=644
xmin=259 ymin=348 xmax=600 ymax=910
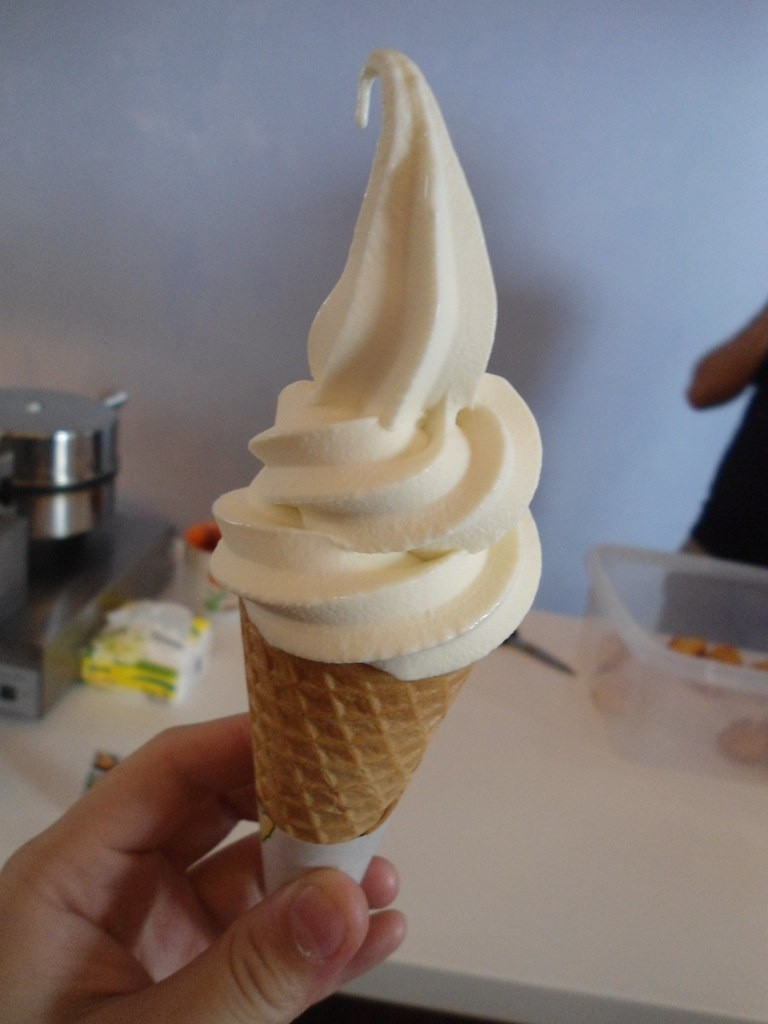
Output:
xmin=204 ymin=51 xmax=544 ymax=847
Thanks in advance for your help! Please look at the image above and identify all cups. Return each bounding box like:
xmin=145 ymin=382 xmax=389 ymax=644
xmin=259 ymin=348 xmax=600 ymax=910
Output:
xmin=174 ymin=539 xmax=236 ymax=610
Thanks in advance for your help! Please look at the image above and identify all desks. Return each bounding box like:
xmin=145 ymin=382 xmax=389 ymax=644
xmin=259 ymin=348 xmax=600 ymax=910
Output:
xmin=20 ymin=580 xmax=767 ymax=1024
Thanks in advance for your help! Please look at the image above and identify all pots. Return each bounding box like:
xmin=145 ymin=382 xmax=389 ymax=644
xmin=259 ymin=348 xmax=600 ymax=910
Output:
xmin=0 ymin=384 xmax=129 ymax=540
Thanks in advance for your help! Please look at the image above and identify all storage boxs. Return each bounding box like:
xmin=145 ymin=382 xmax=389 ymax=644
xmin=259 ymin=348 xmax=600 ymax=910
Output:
xmin=578 ymin=545 xmax=768 ymax=791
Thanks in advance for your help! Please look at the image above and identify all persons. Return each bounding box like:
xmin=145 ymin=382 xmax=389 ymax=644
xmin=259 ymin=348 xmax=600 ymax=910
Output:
xmin=659 ymin=301 xmax=768 ymax=649
xmin=1 ymin=709 xmax=410 ymax=1024
